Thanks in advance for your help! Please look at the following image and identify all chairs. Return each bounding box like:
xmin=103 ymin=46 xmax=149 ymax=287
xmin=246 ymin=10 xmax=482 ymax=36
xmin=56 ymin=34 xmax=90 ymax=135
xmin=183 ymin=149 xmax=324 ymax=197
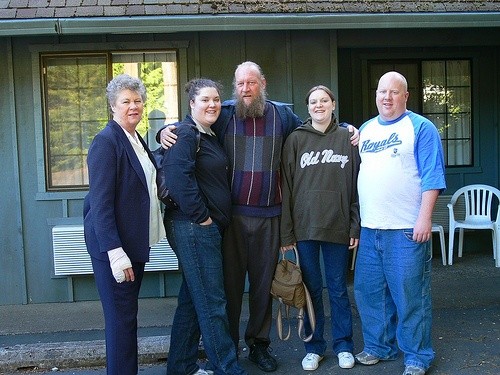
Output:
xmin=431 ymin=184 xmax=500 ymax=267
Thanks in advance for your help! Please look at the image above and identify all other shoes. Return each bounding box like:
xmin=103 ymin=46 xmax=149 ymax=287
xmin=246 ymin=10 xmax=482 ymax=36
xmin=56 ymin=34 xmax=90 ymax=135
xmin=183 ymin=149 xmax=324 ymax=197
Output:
xmin=192 ymin=369 xmax=214 ymax=375
xmin=248 ymin=343 xmax=278 ymax=371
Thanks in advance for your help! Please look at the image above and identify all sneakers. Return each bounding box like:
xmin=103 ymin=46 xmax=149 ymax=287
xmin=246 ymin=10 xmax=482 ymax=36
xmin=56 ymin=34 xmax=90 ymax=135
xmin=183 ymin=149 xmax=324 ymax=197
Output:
xmin=354 ymin=351 xmax=380 ymax=366
xmin=301 ymin=353 xmax=324 ymax=371
xmin=402 ymin=365 xmax=426 ymax=375
xmin=337 ymin=352 xmax=355 ymax=369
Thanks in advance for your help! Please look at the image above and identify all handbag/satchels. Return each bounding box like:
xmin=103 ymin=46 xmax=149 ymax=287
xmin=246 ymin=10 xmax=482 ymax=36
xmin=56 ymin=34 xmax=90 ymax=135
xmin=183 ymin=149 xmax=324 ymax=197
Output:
xmin=156 ymin=167 xmax=178 ymax=211
xmin=270 ymin=245 xmax=306 ymax=308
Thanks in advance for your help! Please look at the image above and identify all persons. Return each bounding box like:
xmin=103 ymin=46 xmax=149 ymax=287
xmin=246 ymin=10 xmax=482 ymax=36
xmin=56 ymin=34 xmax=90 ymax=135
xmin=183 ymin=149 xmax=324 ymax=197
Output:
xmin=280 ymin=85 xmax=362 ymax=371
xmin=353 ymin=71 xmax=446 ymax=375
xmin=163 ymin=78 xmax=232 ymax=375
xmin=155 ymin=61 xmax=360 ymax=374
xmin=83 ymin=74 xmax=166 ymax=375
